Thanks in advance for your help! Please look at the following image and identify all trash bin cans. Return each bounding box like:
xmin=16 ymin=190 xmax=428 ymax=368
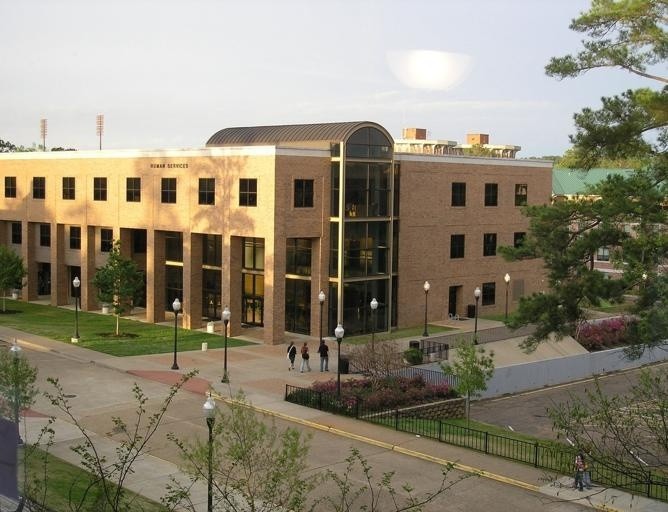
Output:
xmin=409 ymin=340 xmax=419 ymax=350
xmin=339 ymin=357 xmax=349 ymax=373
xmin=468 ymin=304 xmax=475 ymax=318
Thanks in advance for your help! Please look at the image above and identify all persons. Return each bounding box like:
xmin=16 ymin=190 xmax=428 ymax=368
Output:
xmin=582 ymin=456 xmax=593 ymax=488
xmin=299 ymin=341 xmax=312 ymax=373
xmin=286 ymin=341 xmax=297 ymax=371
xmin=572 ymin=453 xmax=584 ymax=491
xmin=317 ymin=339 xmax=329 ymax=372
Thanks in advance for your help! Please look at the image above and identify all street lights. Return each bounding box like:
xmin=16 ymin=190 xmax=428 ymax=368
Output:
xmin=72 ymin=276 xmax=82 ymax=339
xmin=335 ymin=323 xmax=345 ymax=398
xmin=318 ymin=290 xmax=325 ymax=351
xmin=172 ymin=298 xmax=181 ymax=370
xmin=504 ymin=272 xmax=510 ymax=319
xmin=221 ymin=307 xmax=231 ymax=383
xmin=369 ymin=298 xmax=378 ymax=355
xmin=472 ymin=286 xmax=481 ymax=343
xmin=201 ymin=393 xmax=218 ymax=511
xmin=10 ymin=335 xmax=24 ymax=444
xmin=422 ymin=279 xmax=430 ymax=337
